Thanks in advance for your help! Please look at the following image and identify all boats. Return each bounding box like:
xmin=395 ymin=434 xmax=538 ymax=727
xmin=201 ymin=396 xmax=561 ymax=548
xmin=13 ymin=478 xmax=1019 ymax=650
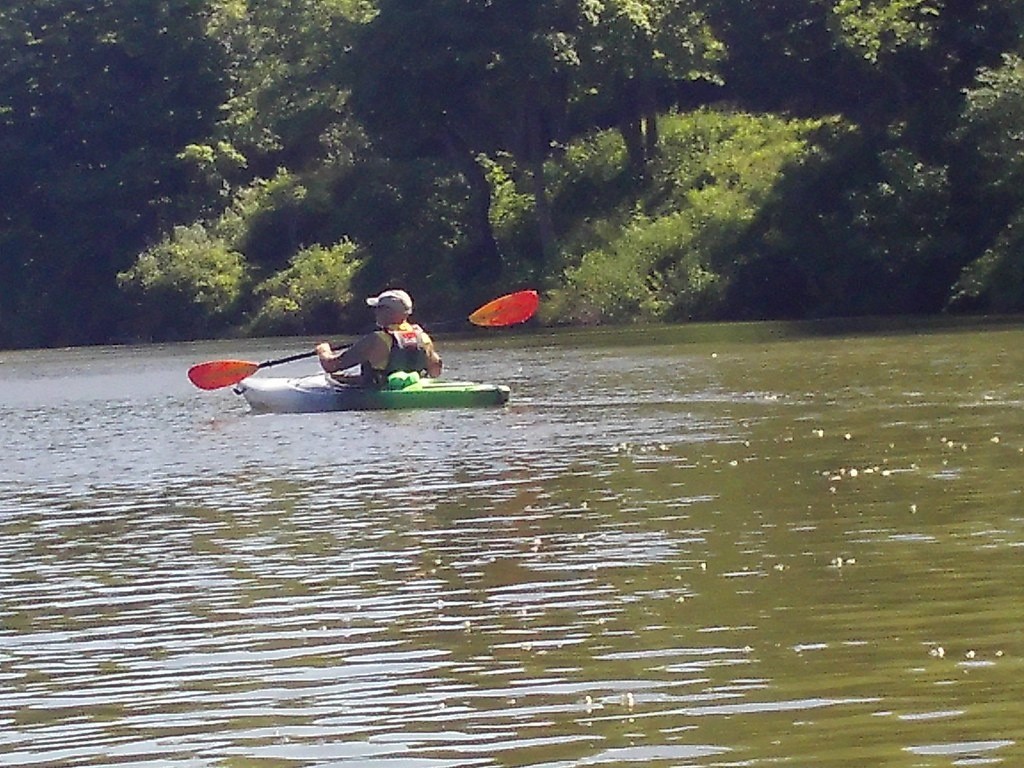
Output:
xmin=236 ymin=371 xmax=512 ymax=412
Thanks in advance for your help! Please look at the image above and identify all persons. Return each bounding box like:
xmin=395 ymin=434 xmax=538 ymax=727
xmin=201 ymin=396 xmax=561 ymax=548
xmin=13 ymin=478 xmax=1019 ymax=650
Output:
xmin=316 ymin=290 xmax=442 ymax=388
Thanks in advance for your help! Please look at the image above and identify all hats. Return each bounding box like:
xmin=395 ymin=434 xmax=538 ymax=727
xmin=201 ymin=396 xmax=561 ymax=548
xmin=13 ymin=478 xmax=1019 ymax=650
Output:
xmin=365 ymin=289 xmax=412 ymax=314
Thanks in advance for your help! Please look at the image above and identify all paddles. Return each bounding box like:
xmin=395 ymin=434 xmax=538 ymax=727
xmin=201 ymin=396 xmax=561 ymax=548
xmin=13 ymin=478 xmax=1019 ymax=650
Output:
xmin=186 ymin=289 xmax=541 ymax=393
